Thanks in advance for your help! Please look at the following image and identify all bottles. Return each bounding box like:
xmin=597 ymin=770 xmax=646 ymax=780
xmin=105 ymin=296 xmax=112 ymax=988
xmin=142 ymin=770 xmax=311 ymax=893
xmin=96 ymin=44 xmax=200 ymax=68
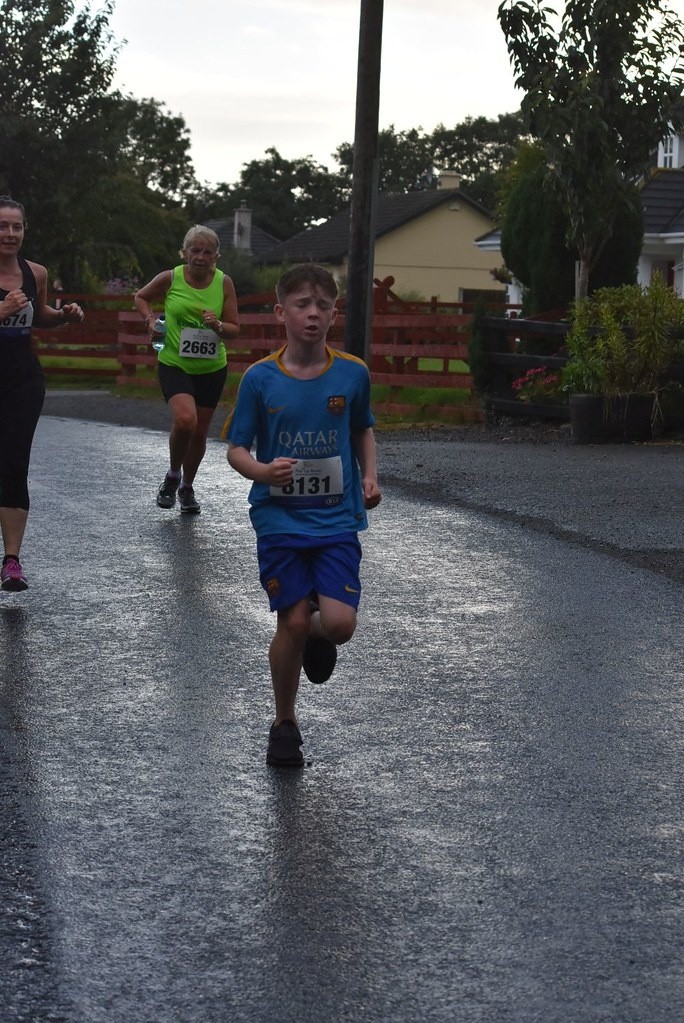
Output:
xmin=151 ymin=314 xmax=166 ymax=351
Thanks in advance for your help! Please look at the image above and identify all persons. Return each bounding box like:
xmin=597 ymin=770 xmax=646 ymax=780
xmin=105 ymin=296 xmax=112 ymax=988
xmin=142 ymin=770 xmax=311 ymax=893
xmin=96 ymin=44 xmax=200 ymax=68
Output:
xmin=0 ymin=194 xmax=87 ymax=592
xmin=221 ymin=265 xmax=382 ymax=768
xmin=134 ymin=225 xmax=240 ymax=514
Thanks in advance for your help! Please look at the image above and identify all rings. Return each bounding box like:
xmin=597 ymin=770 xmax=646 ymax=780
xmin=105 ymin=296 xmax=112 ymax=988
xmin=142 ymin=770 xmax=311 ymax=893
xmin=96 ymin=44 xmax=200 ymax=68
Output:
xmin=208 ymin=317 xmax=211 ymax=320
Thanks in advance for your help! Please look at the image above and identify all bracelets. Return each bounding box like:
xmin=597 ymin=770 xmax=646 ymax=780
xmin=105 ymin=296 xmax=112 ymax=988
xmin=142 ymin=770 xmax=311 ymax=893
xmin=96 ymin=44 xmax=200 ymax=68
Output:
xmin=145 ymin=314 xmax=155 ymax=323
xmin=58 ymin=310 xmax=70 ymax=325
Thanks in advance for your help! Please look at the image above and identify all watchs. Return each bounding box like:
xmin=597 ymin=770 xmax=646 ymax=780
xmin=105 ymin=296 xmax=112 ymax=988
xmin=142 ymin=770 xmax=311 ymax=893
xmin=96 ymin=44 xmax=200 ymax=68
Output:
xmin=214 ymin=320 xmax=223 ymax=333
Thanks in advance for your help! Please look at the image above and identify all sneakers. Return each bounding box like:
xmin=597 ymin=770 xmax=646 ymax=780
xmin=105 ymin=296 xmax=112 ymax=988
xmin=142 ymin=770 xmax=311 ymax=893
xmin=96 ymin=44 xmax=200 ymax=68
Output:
xmin=175 ymin=486 xmax=201 ymax=513
xmin=1 ymin=559 xmax=28 ymax=592
xmin=156 ymin=472 xmax=181 ymax=508
xmin=301 ymin=600 xmax=337 ymax=683
xmin=265 ymin=718 xmax=305 ymax=765
